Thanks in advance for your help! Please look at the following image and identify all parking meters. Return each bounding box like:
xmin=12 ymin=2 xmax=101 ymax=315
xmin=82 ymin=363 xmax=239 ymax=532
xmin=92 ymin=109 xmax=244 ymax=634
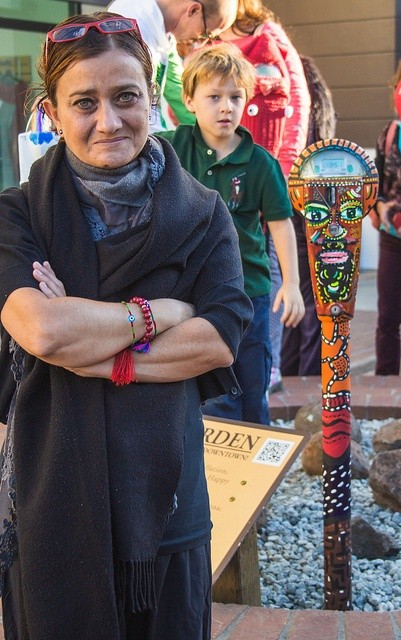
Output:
xmin=289 ymin=138 xmax=379 ymax=610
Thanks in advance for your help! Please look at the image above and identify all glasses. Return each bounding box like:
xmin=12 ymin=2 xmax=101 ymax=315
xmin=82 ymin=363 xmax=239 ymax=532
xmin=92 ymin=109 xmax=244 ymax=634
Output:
xmin=45 ymin=16 xmax=144 ymax=65
xmin=193 ymin=0 xmax=221 ymax=40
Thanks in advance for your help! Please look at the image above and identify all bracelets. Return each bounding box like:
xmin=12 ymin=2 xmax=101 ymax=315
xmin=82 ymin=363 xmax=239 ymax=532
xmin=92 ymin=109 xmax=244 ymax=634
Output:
xmin=121 ymin=295 xmax=159 ymax=347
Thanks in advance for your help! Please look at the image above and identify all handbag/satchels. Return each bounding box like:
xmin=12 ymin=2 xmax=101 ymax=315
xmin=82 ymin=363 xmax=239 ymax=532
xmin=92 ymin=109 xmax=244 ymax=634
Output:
xmin=18 ymin=106 xmax=61 ymax=190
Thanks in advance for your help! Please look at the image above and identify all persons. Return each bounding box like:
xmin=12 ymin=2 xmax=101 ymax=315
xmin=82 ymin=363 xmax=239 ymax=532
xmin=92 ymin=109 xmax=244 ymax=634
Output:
xmin=165 ymin=0 xmax=312 ymax=393
xmin=0 ymin=10 xmax=255 ymax=640
xmin=148 ymin=43 xmax=307 ymax=426
xmin=280 ymin=50 xmax=337 ymax=376
xmin=105 ymin=0 xmax=239 ymax=137
xmin=369 ymin=80 xmax=401 ymax=376
